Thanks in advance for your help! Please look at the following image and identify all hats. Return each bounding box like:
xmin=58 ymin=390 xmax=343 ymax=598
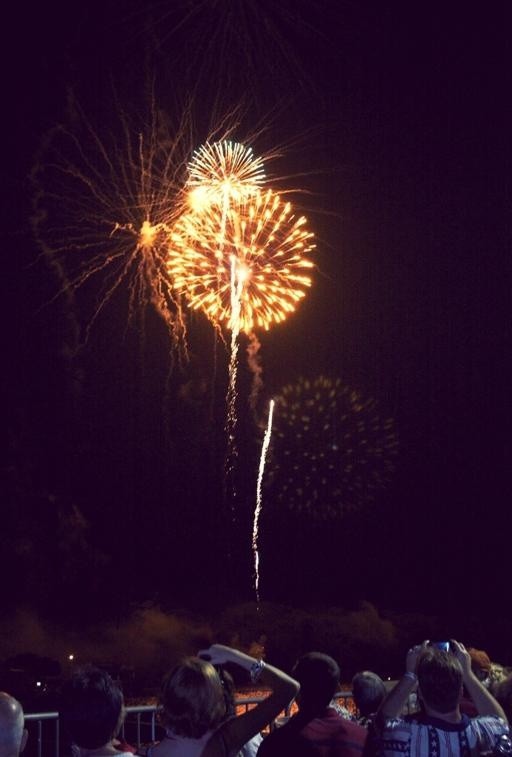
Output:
xmin=469 ymin=647 xmax=491 ymax=673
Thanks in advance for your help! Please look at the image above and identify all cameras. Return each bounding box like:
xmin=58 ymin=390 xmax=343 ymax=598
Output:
xmin=430 ymin=641 xmax=450 ymax=654
xmin=494 ymin=734 xmax=512 ymax=757
xmin=200 ymin=654 xmax=224 ymax=682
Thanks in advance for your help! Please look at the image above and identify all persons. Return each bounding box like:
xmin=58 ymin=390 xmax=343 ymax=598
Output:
xmin=0 ymin=638 xmax=510 ymax=755
